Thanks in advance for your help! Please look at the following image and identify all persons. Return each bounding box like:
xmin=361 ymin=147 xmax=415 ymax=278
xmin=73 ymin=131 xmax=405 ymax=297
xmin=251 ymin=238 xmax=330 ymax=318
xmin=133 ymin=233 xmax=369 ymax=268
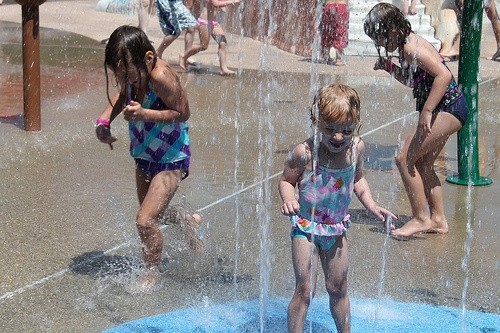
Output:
xmin=278 ymin=84 xmax=397 ymax=333
xmin=364 ymin=3 xmax=468 ymax=238
xmin=137 ymin=0 xmax=500 ymax=75
xmin=95 ymin=25 xmax=202 ymax=294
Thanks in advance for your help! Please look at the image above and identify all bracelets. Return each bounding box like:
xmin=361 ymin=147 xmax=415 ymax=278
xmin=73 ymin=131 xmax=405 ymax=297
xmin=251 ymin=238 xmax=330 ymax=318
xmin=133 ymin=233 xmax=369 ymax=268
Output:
xmin=95 ymin=119 xmax=110 ymax=126
